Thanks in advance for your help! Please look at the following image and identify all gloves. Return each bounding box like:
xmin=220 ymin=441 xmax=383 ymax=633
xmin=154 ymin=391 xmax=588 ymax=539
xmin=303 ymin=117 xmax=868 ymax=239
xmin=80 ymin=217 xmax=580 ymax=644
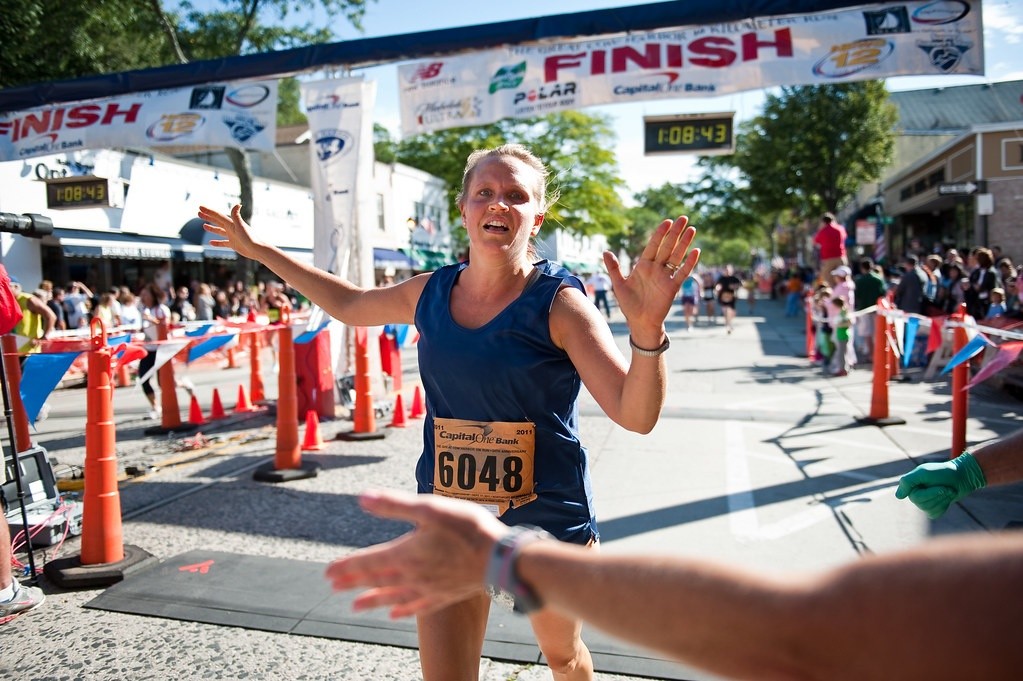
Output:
xmin=896 ymin=452 xmax=987 ymax=519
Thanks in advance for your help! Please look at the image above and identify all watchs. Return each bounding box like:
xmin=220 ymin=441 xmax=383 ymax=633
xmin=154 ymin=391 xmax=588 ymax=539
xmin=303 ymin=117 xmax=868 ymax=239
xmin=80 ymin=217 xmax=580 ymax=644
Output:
xmin=482 ymin=525 xmax=558 ymax=615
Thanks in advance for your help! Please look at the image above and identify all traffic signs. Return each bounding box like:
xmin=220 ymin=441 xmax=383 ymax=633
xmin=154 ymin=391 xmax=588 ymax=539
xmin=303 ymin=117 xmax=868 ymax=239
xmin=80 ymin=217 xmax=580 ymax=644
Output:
xmin=936 ymin=179 xmax=987 ymax=198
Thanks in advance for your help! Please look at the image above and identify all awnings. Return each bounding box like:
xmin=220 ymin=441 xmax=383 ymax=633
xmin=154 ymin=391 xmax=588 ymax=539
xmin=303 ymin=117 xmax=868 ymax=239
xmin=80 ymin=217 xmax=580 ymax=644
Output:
xmin=50 ymin=227 xmax=237 ymax=262
xmin=398 ymin=249 xmax=449 ymax=271
xmin=372 ymin=248 xmax=421 ymax=271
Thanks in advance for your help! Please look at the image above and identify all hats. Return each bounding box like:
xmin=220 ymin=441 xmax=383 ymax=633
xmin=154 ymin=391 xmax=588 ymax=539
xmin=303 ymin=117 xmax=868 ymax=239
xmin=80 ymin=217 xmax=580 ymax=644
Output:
xmin=830 ymin=265 xmax=851 ymax=278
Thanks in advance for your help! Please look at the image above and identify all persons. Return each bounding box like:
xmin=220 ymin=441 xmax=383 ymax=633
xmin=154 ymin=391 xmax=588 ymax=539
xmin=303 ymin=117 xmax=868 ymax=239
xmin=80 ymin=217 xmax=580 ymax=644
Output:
xmin=259 ymin=281 xmax=294 ymax=374
xmin=196 ymin=143 xmax=701 ymax=681
xmin=132 ymin=280 xmax=197 ymax=421
xmin=770 ymin=210 xmax=1023 ymax=399
xmin=323 ymin=488 xmax=1023 ymax=680
xmin=894 ymin=427 xmax=1023 ymax=520
xmin=679 ymin=270 xmax=757 ymax=334
xmin=584 ymin=267 xmax=614 ymax=318
xmin=116 ymin=279 xmax=310 ymax=325
xmin=0 ymin=262 xmax=46 ymax=628
xmin=6 ymin=273 xmax=122 ymax=422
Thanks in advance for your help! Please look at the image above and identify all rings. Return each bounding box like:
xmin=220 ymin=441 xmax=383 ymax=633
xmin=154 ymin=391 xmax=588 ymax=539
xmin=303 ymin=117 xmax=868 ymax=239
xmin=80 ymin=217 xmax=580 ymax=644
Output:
xmin=665 ymin=262 xmax=678 ymax=272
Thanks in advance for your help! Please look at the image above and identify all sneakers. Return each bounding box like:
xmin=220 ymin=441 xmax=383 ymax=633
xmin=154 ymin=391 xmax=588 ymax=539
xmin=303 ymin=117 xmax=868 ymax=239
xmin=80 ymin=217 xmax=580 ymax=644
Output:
xmin=0 ymin=576 xmax=45 ymax=624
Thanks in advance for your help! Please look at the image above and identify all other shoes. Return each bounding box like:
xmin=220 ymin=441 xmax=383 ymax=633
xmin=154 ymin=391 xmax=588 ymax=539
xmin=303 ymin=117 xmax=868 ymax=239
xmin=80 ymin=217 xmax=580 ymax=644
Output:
xmin=144 ymin=408 xmax=162 ymax=419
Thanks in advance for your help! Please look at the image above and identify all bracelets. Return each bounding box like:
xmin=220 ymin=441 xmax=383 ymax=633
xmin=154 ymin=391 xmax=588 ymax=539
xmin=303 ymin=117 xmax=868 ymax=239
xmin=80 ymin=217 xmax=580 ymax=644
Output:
xmin=629 ymin=330 xmax=671 ymax=358
